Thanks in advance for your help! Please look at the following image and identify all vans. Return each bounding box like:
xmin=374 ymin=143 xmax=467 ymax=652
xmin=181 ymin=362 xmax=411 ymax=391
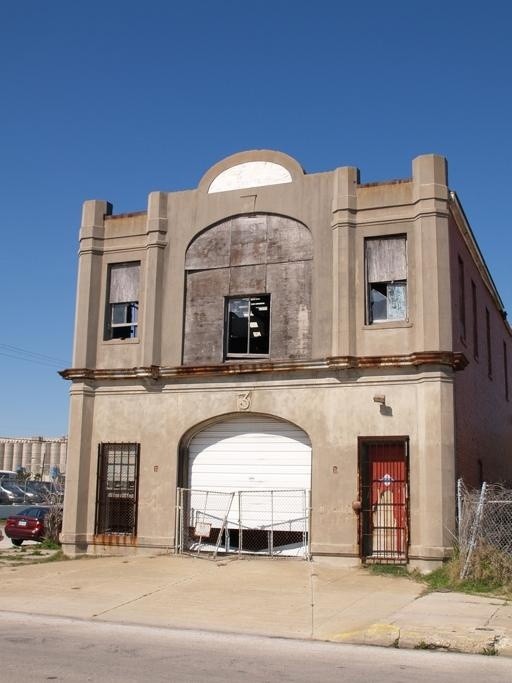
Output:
xmin=0 ymin=470 xmax=64 ymax=505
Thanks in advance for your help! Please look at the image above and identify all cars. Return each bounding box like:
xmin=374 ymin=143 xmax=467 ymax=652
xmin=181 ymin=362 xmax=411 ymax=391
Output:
xmin=5 ymin=504 xmax=62 ymax=547
